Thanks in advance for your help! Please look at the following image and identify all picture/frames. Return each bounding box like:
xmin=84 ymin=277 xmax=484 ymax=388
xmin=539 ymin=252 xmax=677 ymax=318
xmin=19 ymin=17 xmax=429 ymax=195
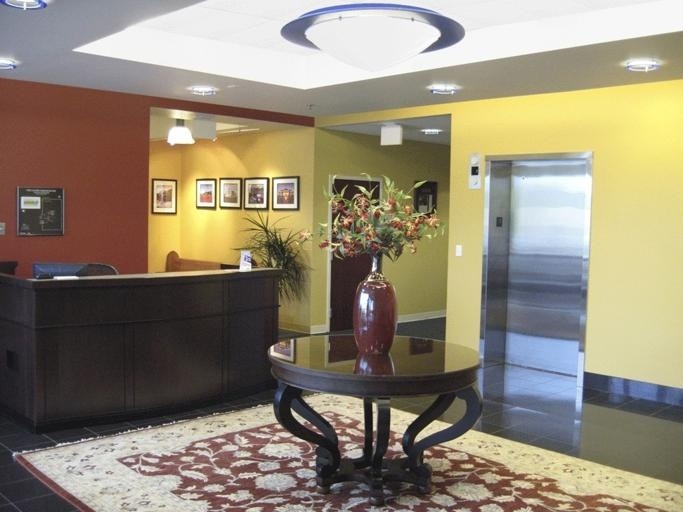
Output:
xmin=195 ymin=178 xmax=216 ymax=209
xmin=407 ymin=336 xmax=434 ymax=357
xmin=413 ymin=179 xmax=438 ymax=220
xmin=272 ymin=176 xmax=299 ymax=210
xmin=268 ymin=337 xmax=295 ymax=364
xmin=151 ymin=178 xmax=177 ymax=214
xmin=243 ymin=177 xmax=269 ymax=210
xmin=218 ymin=177 xmax=242 ymax=209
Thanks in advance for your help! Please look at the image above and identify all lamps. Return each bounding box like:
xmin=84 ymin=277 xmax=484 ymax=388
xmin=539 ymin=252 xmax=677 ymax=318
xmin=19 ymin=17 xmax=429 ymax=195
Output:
xmin=279 ymin=3 xmax=464 ymax=73
xmin=166 ymin=118 xmax=195 ymax=146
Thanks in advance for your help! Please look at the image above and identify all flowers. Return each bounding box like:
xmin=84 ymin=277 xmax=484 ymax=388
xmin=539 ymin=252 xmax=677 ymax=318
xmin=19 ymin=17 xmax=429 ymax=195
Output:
xmin=300 ymin=171 xmax=447 ymax=264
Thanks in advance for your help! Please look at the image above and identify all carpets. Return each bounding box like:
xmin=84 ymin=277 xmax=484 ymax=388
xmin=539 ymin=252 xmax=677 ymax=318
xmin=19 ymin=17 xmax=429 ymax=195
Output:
xmin=12 ymin=390 xmax=682 ymax=512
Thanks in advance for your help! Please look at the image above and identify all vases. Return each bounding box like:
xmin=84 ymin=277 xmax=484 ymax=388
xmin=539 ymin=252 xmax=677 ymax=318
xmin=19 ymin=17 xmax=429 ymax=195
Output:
xmin=353 ymin=351 xmax=395 ymax=376
xmin=352 ymin=252 xmax=396 ymax=355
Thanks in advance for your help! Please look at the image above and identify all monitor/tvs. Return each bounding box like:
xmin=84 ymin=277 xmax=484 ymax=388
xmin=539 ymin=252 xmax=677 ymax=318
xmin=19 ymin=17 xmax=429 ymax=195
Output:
xmin=33 ymin=264 xmax=88 ymax=279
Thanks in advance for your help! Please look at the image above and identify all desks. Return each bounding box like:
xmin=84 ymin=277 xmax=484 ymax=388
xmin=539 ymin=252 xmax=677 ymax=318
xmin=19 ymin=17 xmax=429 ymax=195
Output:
xmin=265 ymin=334 xmax=483 ymax=507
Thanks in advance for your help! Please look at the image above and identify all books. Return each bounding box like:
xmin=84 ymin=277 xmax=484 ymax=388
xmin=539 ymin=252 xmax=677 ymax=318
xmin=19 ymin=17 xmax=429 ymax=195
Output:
xmin=54 ymin=275 xmax=79 ymax=280
